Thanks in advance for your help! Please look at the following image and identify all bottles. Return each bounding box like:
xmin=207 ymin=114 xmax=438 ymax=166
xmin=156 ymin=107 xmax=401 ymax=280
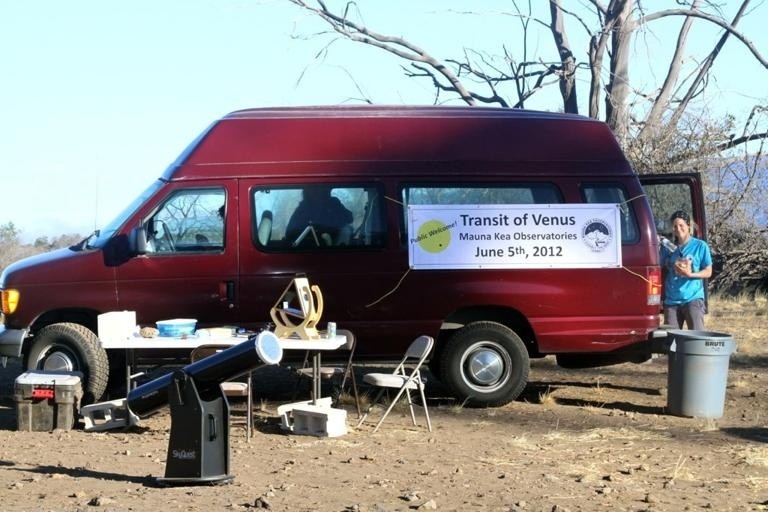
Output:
xmin=660 ymin=236 xmax=678 ymax=252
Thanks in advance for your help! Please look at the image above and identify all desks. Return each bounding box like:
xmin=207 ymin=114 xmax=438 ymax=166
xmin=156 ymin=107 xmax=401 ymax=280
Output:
xmin=102 ymin=324 xmax=348 ymax=431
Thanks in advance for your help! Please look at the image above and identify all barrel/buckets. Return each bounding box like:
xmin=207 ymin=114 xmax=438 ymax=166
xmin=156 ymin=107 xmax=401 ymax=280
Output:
xmin=667 ymin=328 xmax=736 ymax=420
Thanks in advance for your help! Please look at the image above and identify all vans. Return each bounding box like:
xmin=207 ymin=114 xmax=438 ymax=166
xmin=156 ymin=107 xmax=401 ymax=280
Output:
xmin=0 ymin=104 xmax=707 ymax=408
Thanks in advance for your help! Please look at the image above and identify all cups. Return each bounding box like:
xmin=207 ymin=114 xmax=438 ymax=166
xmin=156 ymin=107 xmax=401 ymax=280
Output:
xmin=327 ymin=322 xmax=336 ymax=337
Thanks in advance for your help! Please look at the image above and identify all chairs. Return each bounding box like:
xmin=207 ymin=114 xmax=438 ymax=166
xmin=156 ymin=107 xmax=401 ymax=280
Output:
xmin=295 ymin=329 xmax=362 ymax=419
xmin=257 ymin=210 xmax=272 ymax=245
xmin=188 ymin=343 xmax=256 ymax=442
xmin=357 ymin=333 xmax=433 ymax=434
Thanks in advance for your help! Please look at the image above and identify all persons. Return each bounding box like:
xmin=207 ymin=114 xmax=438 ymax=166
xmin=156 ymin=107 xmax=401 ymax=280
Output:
xmin=660 ymin=211 xmax=714 ymax=332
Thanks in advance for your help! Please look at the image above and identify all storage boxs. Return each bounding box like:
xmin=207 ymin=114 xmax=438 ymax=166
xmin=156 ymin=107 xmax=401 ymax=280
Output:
xmin=156 ymin=318 xmax=197 ymax=338
xmin=14 ymin=367 xmax=83 ymax=434
xmin=97 ymin=311 xmax=137 ymax=342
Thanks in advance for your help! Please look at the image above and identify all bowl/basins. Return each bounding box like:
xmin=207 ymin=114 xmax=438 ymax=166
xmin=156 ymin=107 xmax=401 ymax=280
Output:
xmin=158 ymin=319 xmax=197 ymax=337
